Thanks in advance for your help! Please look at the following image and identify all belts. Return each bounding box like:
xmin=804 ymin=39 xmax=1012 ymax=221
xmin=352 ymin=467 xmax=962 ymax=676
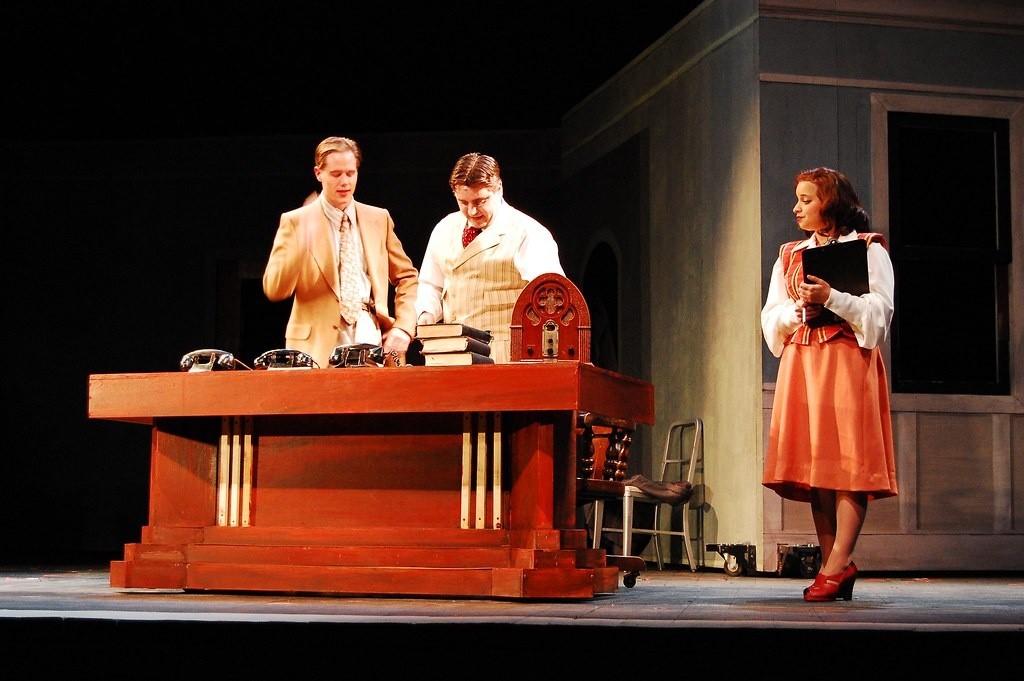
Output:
xmin=362 ymin=305 xmax=371 ymax=313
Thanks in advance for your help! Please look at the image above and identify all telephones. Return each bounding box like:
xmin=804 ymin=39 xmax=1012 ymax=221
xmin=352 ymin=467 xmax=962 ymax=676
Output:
xmin=178 ymin=349 xmax=236 ymax=372
xmin=254 ymin=349 xmax=321 ymax=369
xmin=328 ymin=343 xmax=397 ymax=367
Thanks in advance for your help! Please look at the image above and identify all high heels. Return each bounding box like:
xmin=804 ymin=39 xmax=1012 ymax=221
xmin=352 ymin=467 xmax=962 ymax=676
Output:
xmin=803 ymin=561 xmax=858 ymax=604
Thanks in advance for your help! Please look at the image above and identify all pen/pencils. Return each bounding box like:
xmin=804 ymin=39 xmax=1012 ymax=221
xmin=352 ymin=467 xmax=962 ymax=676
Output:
xmin=803 ymin=308 xmax=806 ymax=323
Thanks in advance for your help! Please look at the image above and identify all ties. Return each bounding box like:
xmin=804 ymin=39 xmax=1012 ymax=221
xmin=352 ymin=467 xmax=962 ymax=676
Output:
xmin=463 ymin=225 xmax=483 ymax=248
xmin=339 ymin=214 xmax=367 ymax=325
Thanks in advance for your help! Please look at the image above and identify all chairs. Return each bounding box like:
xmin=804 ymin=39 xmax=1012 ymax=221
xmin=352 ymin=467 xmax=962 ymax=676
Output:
xmin=592 ymin=418 xmax=701 ymax=571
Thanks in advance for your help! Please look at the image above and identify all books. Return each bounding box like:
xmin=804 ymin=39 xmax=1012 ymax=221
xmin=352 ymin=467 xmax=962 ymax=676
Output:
xmin=414 ymin=323 xmax=495 ymax=367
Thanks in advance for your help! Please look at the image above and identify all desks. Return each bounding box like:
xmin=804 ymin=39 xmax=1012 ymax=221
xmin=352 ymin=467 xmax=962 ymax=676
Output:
xmin=89 ymin=363 xmax=654 ymax=602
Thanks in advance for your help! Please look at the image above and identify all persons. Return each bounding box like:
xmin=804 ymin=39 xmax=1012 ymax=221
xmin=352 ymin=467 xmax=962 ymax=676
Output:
xmin=760 ymin=167 xmax=899 ymax=601
xmin=415 ymin=152 xmax=567 ymax=364
xmin=262 ymin=136 xmax=419 ymax=369
